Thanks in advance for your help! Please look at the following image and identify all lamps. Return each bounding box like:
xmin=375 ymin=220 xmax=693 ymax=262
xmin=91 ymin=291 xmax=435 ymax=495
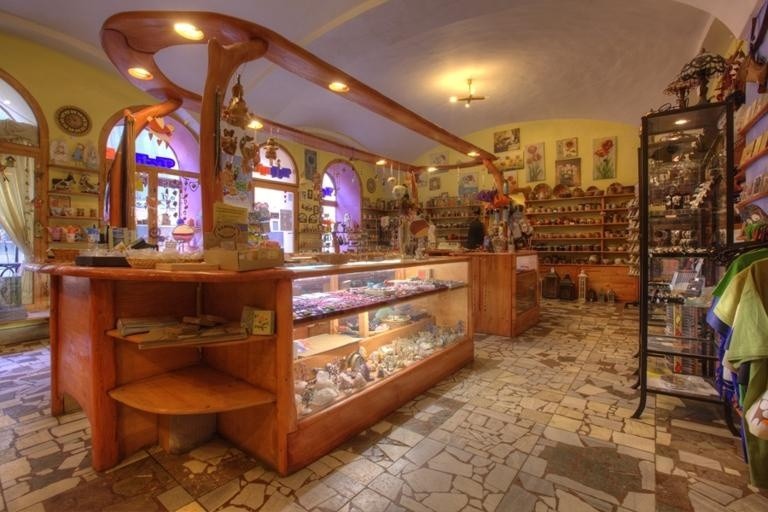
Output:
xmin=449 ymin=78 xmax=486 ymax=107
xmin=664 ymin=74 xmax=696 ymax=108
xmin=681 ymin=48 xmax=731 ymax=104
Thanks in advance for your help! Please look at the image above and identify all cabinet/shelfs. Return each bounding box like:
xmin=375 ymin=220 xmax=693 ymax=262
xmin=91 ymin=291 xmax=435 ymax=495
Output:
xmin=24 ymin=99 xmax=768 ymax=473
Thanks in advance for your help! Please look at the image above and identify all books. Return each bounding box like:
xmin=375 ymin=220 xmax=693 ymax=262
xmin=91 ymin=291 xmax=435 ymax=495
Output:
xmin=117 ymin=305 xmax=277 ymax=352
